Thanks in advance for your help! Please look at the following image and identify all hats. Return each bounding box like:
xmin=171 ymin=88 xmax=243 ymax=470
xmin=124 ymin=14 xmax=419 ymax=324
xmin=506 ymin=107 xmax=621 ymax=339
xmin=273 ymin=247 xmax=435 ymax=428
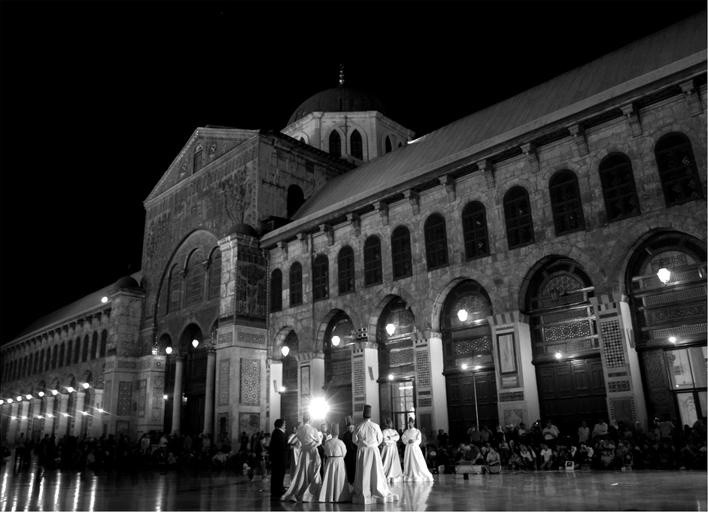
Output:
xmin=303 ymin=412 xmax=309 ymax=419
xmin=386 ymin=417 xmax=391 ymax=424
xmin=345 ymin=416 xmax=353 ymax=426
xmin=331 ymin=424 xmax=339 ymax=435
xmin=363 ymin=406 xmax=371 ymax=417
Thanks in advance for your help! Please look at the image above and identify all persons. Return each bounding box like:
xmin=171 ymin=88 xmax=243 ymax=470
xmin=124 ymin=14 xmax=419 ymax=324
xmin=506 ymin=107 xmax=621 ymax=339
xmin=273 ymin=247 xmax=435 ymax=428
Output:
xmin=380 ymin=417 xmax=403 ymax=483
xmin=281 ymin=405 xmax=399 ymax=504
xmin=2 ymin=431 xmax=272 ymax=493
xmin=380 ymin=415 xmax=707 ymax=479
xmin=401 ymin=417 xmax=434 ymax=481
xmin=267 ymin=419 xmax=292 ymax=510
